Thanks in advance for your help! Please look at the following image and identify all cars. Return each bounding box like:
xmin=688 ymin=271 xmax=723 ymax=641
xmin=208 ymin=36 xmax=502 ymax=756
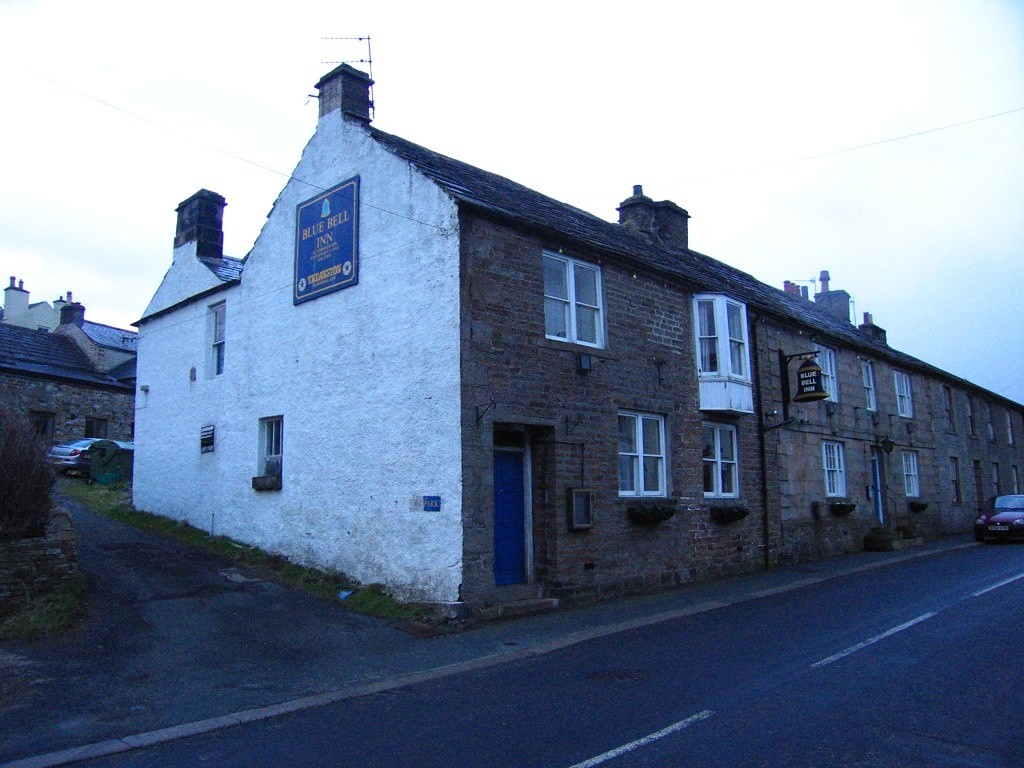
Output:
xmin=44 ymin=437 xmax=134 ymax=476
xmin=974 ymin=494 xmax=1024 ymax=543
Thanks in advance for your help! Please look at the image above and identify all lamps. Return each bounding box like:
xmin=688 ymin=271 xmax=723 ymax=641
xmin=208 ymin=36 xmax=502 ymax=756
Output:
xmin=876 ymin=435 xmax=895 ymax=455
